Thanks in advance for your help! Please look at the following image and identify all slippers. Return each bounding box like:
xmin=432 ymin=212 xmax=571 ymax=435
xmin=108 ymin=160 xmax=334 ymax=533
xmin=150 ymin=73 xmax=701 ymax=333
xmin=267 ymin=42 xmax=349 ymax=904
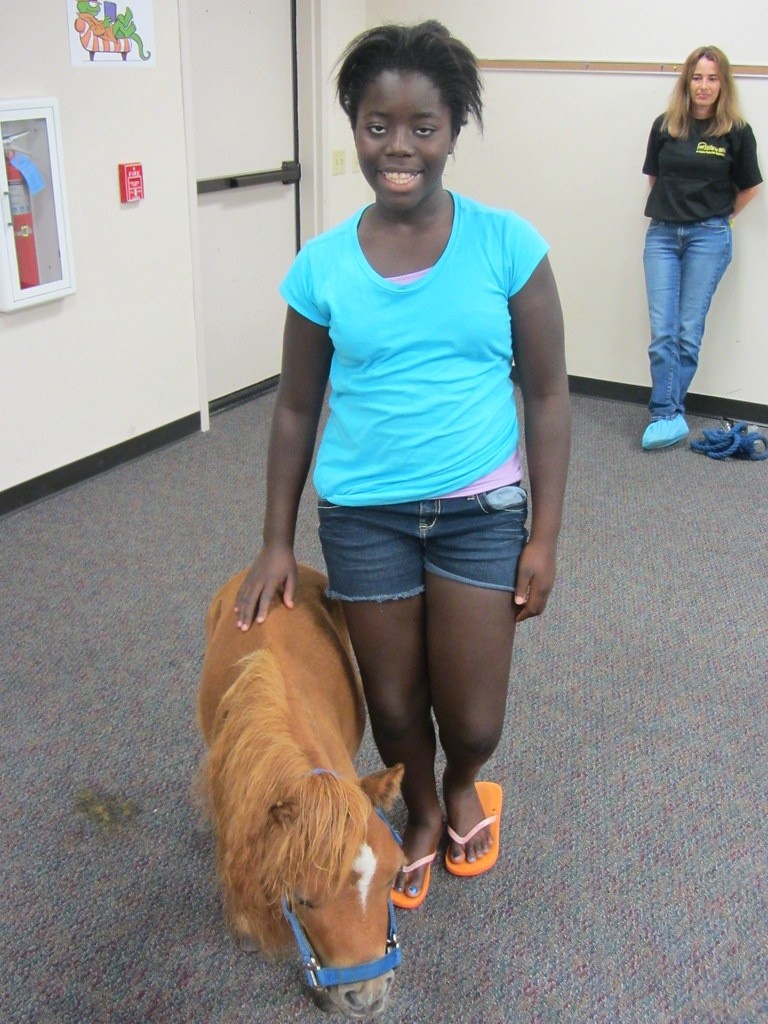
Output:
xmin=445 ymin=782 xmax=504 ymax=876
xmin=389 ymin=852 xmax=438 ymax=909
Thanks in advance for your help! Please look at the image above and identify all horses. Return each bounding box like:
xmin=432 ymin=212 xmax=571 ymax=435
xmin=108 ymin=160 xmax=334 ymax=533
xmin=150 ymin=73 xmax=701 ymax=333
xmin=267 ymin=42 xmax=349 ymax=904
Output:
xmin=187 ymin=563 xmax=405 ymax=1024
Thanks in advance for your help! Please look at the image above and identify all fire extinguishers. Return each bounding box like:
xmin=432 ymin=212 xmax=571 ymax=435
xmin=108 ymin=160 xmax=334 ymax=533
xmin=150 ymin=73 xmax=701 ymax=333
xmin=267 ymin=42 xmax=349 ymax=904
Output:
xmin=2 ymin=129 xmax=42 ymax=288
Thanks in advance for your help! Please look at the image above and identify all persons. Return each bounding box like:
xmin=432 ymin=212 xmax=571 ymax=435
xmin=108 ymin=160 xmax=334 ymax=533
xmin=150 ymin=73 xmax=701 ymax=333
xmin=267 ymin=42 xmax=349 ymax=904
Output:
xmin=234 ymin=19 xmax=572 ymax=910
xmin=641 ymin=45 xmax=763 ymax=451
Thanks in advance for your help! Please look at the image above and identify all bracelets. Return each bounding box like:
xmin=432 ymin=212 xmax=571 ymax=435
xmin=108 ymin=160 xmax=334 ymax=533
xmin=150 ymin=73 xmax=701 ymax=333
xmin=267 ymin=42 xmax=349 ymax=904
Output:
xmin=729 ymin=213 xmax=734 ymax=229
